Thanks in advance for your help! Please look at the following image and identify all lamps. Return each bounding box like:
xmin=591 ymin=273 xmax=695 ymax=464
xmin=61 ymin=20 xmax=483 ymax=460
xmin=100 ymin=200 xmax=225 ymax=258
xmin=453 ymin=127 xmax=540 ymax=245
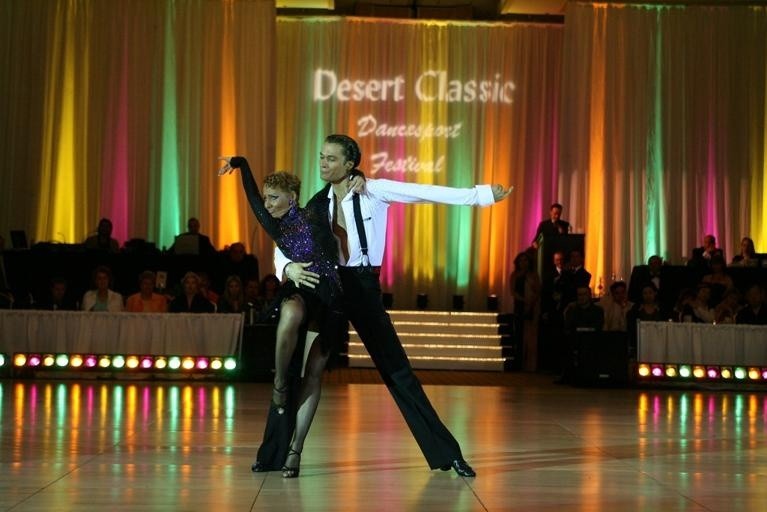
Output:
xmin=452 ymin=295 xmax=464 ymax=313
xmin=415 ymin=292 xmax=427 ymax=312
xmin=634 ymin=362 xmax=766 ymax=391
xmin=0 ymin=350 xmax=241 ymax=370
xmin=381 ymin=290 xmax=395 ymax=310
xmin=487 ymin=293 xmax=500 ymax=312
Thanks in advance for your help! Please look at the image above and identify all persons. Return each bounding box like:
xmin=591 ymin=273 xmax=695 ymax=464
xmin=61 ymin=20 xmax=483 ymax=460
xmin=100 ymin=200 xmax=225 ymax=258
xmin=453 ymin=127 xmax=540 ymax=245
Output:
xmin=212 ymin=150 xmax=367 ymax=478
xmin=31 ymin=216 xmax=278 ymax=322
xmin=270 ymin=133 xmax=517 ymax=478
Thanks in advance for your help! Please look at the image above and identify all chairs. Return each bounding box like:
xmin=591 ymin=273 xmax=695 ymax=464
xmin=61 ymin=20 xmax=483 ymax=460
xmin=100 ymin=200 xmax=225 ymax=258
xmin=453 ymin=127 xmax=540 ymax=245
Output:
xmin=561 ymin=242 xmax=766 ymax=331
xmin=0 ymin=230 xmax=286 ymax=330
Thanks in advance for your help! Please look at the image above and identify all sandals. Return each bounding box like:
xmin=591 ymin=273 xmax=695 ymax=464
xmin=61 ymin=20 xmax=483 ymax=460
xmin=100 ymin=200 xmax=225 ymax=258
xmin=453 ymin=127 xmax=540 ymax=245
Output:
xmin=272 ymin=381 xmax=290 ymax=416
xmin=282 ymin=445 xmax=303 ymax=479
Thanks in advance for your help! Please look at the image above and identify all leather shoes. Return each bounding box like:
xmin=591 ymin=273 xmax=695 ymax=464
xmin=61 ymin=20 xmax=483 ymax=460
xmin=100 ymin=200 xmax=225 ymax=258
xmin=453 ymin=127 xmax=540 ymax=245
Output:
xmin=251 ymin=453 xmax=278 ymax=472
xmin=449 ymin=445 xmax=477 ymax=478
